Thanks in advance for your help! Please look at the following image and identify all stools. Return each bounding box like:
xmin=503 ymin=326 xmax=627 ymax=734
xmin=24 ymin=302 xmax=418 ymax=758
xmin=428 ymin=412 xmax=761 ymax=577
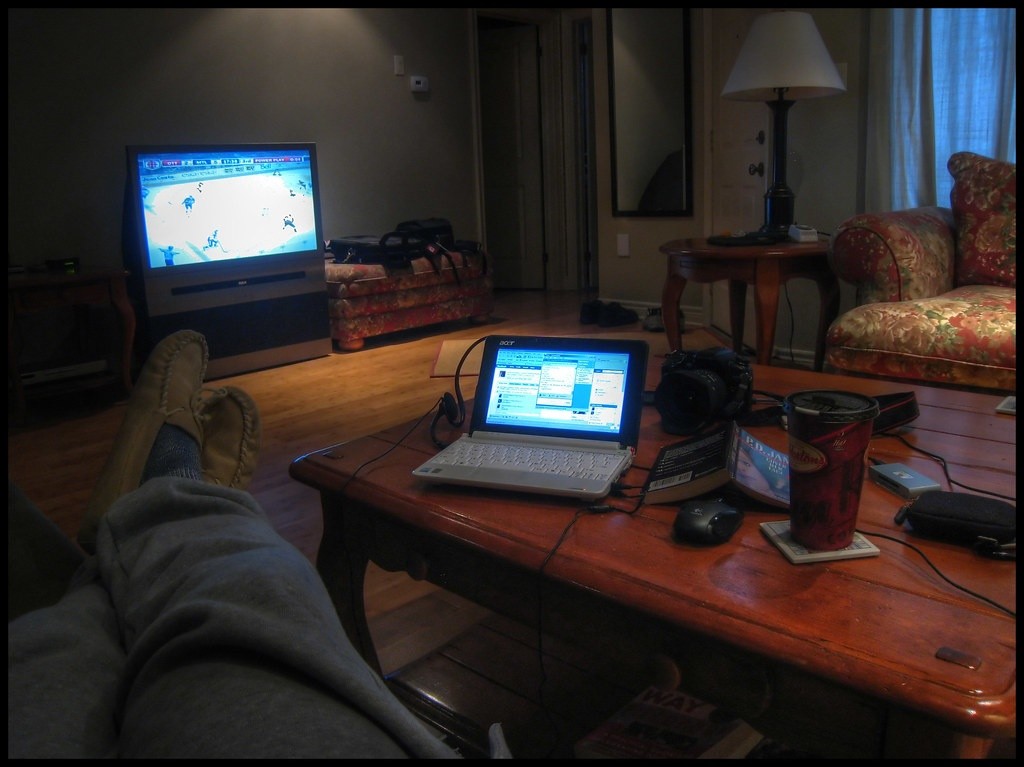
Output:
xmin=323 ymin=251 xmax=496 ymax=351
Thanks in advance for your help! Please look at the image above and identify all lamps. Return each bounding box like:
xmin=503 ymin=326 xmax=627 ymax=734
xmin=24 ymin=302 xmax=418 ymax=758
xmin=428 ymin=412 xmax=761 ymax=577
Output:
xmin=706 ymin=10 xmax=848 ymax=249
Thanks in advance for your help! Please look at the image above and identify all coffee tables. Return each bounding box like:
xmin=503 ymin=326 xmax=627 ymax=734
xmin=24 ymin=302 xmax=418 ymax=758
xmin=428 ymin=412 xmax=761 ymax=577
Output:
xmin=289 ymin=358 xmax=1017 ymax=758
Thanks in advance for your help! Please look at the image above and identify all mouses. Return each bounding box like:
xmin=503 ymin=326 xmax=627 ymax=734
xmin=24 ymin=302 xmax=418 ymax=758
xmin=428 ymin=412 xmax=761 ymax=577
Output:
xmin=673 ymin=497 xmax=744 ymax=547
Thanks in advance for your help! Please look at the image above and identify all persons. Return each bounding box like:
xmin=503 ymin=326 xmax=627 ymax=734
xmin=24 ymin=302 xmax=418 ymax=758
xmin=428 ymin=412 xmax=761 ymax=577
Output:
xmin=7 ymin=329 xmax=466 ymax=759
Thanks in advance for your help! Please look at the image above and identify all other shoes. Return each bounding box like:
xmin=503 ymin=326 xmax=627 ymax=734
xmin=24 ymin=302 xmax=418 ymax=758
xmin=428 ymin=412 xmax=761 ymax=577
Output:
xmin=581 ymin=299 xmax=606 ymax=325
xmin=598 ymin=302 xmax=639 ymax=327
xmin=642 ymin=306 xmax=685 ymax=334
xmin=76 ymin=329 xmax=208 ymax=555
xmin=193 ymin=385 xmax=260 ymax=492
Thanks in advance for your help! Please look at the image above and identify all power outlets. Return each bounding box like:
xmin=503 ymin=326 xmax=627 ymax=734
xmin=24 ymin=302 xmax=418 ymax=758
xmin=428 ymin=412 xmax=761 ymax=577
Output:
xmin=617 ymin=233 xmax=630 ymax=258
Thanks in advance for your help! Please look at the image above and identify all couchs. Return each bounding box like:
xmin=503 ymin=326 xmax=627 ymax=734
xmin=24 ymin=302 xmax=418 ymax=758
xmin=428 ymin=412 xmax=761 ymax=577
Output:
xmin=825 ymin=150 xmax=1016 ymax=395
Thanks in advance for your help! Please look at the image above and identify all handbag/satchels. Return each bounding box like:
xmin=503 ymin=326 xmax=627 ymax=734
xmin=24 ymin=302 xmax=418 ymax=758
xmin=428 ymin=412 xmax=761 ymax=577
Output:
xmin=398 ymin=217 xmax=455 ymax=256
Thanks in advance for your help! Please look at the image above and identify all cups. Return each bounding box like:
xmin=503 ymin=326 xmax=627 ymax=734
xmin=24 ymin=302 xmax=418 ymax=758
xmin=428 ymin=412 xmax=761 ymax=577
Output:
xmin=785 ymin=389 xmax=879 ymax=551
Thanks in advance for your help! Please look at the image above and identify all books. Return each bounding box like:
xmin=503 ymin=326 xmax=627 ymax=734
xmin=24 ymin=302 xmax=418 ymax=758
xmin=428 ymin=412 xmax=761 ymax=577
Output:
xmin=641 ymin=418 xmax=789 ymax=511
xmin=572 ymin=684 xmax=766 ymax=759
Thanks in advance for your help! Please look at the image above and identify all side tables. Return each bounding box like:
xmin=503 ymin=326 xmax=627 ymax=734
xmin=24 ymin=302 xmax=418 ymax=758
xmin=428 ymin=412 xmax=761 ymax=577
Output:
xmin=660 ymin=229 xmax=839 ymax=372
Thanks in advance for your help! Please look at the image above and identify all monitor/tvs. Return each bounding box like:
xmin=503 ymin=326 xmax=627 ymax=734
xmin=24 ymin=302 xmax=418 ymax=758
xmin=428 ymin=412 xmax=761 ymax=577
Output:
xmin=124 ymin=143 xmax=325 ymax=315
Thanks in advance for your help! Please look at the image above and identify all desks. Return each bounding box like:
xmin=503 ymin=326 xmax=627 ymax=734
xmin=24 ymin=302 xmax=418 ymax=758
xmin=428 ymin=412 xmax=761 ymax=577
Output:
xmin=6 ymin=264 xmax=135 ymax=427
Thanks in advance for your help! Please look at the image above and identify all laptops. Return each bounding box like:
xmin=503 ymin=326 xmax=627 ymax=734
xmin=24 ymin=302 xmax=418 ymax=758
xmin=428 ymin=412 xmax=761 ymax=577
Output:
xmin=412 ymin=336 xmax=650 ymax=500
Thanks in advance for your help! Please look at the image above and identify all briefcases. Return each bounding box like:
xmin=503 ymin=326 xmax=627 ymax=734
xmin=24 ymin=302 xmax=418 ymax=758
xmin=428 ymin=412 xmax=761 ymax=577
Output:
xmin=330 ymin=230 xmax=424 ymax=269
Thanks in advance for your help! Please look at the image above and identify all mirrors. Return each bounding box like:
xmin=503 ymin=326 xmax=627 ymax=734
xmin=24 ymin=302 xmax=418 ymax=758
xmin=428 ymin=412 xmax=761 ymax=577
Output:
xmin=606 ymin=7 xmax=693 ymax=218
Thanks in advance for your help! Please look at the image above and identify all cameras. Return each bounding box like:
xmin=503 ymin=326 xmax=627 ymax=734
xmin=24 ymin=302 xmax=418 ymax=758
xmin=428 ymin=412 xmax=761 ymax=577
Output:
xmin=654 ymin=347 xmax=754 ymax=437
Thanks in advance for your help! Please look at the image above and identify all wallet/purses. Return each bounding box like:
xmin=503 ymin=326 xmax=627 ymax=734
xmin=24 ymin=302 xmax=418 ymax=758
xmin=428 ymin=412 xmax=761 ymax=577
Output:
xmin=893 ymin=490 xmax=1017 ymax=546
